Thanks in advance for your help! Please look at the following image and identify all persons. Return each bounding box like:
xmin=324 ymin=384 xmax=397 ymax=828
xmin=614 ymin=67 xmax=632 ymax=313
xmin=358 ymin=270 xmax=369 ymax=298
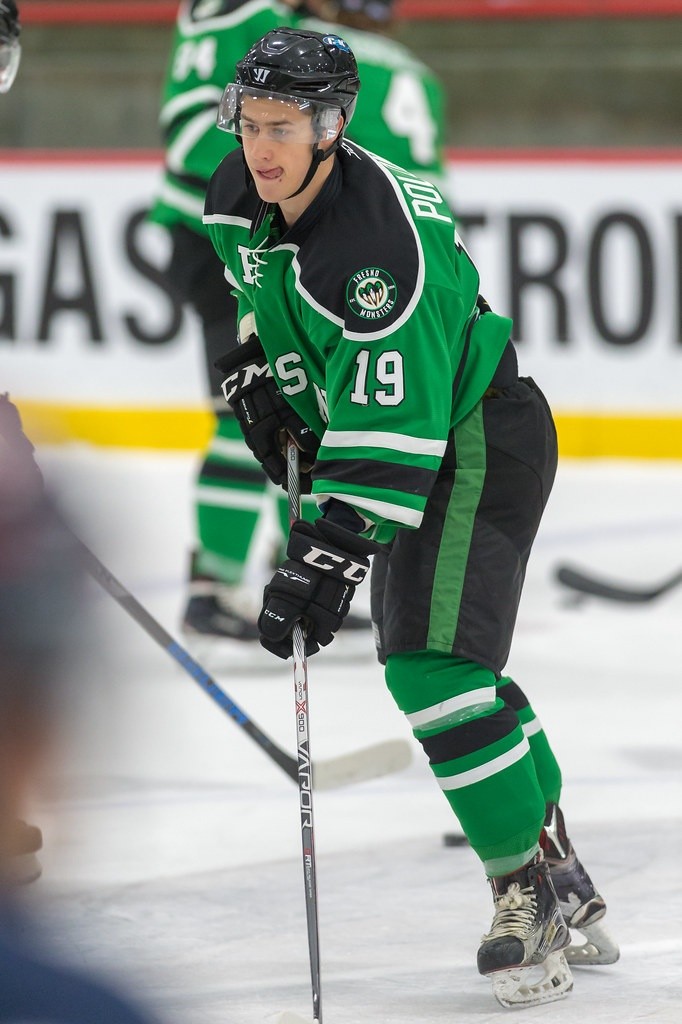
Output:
xmin=202 ymin=28 xmax=623 ymax=1009
xmin=0 ymin=0 xmax=45 ymax=888
xmin=149 ymin=1 xmax=446 ymax=643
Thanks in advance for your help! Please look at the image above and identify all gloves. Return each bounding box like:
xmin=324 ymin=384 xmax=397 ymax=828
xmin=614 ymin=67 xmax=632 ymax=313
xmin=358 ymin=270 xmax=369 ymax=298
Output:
xmin=213 ymin=335 xmax=319 ymax=496
xmin=256 ymin=519 xmax=381 ymax=660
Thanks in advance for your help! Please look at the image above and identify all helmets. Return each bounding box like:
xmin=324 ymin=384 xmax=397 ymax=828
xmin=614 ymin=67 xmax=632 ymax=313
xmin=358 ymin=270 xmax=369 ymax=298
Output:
xmin=236 ymin=27 xmax=361 ymax=110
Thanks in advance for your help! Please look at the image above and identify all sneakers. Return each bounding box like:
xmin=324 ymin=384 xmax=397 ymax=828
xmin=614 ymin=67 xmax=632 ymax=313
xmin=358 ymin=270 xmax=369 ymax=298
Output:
xmin=476 ymin=848 xmax=575 ymax=1010
xmin=538 ymin=803 xmax=622 ymax=966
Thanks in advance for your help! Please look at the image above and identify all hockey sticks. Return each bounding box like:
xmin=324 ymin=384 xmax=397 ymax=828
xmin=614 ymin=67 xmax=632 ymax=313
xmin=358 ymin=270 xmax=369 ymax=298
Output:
xmin=42 ymin=497 xmax=415 ymax=792
xmin=553 ymin=562 xmax=681 ymax=610
xmin=280 ymin=424 xmax=326 ymax=1024
